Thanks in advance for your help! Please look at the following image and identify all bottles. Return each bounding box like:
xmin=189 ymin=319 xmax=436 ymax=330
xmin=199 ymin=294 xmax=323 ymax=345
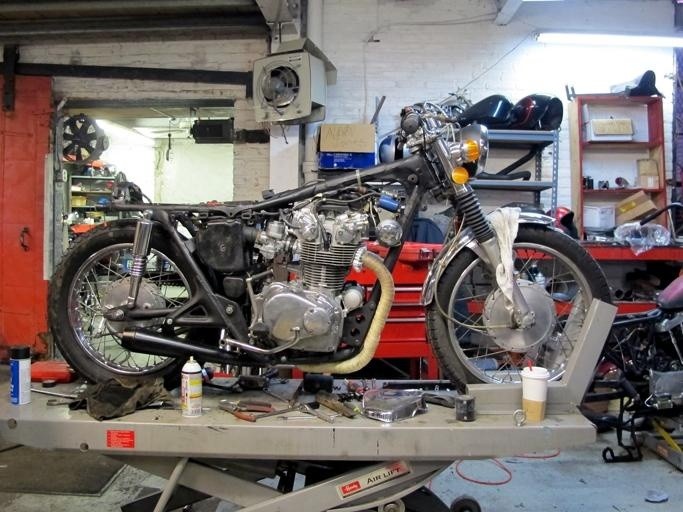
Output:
xmin=583 ymin=176 xmax=593 ymax=190
xmin=180 ymin=356 xmax=204 ymax=417
xmin=515 ymin=260 xmax=546 ymax=288
xmin=122 ymin=248 xmax=134 ymax=273
xmin=9 ymin=344 xmax=31 ymax=405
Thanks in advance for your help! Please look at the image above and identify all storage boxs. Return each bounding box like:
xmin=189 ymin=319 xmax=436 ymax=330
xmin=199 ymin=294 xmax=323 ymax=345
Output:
xmin=317 ymin=123 xmax=378 ymax=173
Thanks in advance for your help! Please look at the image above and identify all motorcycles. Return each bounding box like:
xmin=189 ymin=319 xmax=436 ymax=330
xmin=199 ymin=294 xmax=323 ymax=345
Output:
xmin=524 ymin=201 xmax=682 ymax=434
xmin=47 ymin=89 xmax=616 ymax=401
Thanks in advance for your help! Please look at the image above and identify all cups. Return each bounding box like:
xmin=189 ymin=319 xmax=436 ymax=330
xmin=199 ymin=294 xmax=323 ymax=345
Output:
xmin=520 ymin=366 xmax=550 ymax=424
xmin=456 ymin=395 xmax=475 ymax=422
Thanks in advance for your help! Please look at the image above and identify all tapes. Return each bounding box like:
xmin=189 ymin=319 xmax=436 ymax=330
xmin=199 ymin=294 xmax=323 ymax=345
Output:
xmin=42 ymin=379 xmax=56 ymax=387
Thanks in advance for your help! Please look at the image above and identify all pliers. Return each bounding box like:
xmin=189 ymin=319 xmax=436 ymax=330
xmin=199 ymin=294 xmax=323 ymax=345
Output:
xmin=219 ymin=401 xmax=272 ymax=422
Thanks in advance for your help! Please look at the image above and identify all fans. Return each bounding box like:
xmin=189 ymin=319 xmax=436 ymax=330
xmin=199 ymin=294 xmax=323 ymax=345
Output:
xmin=62 ymin=112 xmax=109 ymax=165
xmin=253 ymin=53 xmax=311 ymax=123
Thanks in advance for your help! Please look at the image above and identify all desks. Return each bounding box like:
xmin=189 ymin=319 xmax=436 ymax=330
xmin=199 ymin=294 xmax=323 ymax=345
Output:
xmin=0 ymin=376 xmax=597 ymax=511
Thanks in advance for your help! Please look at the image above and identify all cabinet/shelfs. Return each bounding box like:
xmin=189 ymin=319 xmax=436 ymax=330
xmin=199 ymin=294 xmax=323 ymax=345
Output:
xmin=66 ymin=175 xmax=124 ymax=225
xmin=567 ymin=93 xmax=671 ymax=243
xmin=468 ymin=126 xmax=560 ymax=232
